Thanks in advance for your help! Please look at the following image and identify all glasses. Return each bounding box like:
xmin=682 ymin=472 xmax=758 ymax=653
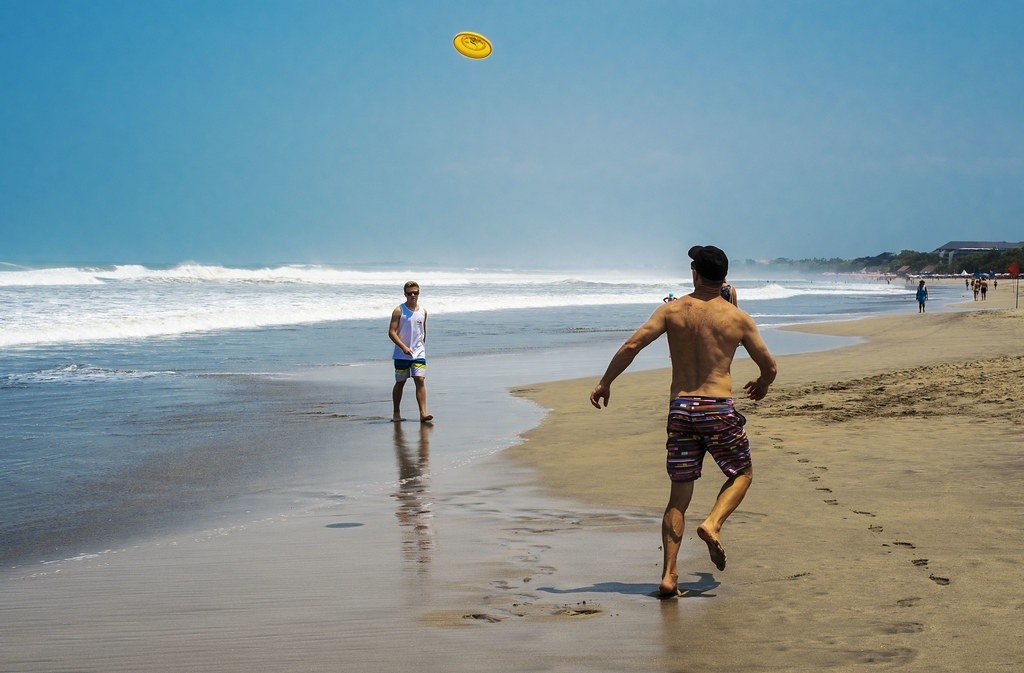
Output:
xmin=405 ymin=290 xmax=419 ymax=296
xmin=690 ymin=261 xmax=697 ymax=271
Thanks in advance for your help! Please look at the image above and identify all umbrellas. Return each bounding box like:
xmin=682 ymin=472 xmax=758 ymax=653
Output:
xmin=908 ymin=270 xmax=1024 ymax=279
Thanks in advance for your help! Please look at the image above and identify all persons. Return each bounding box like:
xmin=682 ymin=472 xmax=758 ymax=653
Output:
xmin=886 ymin=274 xmax=997 ymax=313
xmin=663 ymin=294 xmax=677 ymax=302
xmin=389 ymin=281 xmax=433 ymax=422
xmin=590 ymin=246 xmax=778 ymax=594
xmin=721 ymin=279 xmax=743 ymax=346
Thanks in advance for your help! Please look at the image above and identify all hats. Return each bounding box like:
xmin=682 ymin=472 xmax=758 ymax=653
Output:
xmin=669 ymin=293 xmax=673 ymax=299
xmin=688 ymin=245 xmax=729 ymax=276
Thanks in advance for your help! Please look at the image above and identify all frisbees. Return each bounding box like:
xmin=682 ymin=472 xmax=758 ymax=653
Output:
xmin=452 ymin=31 xmax=494 ymax=59
